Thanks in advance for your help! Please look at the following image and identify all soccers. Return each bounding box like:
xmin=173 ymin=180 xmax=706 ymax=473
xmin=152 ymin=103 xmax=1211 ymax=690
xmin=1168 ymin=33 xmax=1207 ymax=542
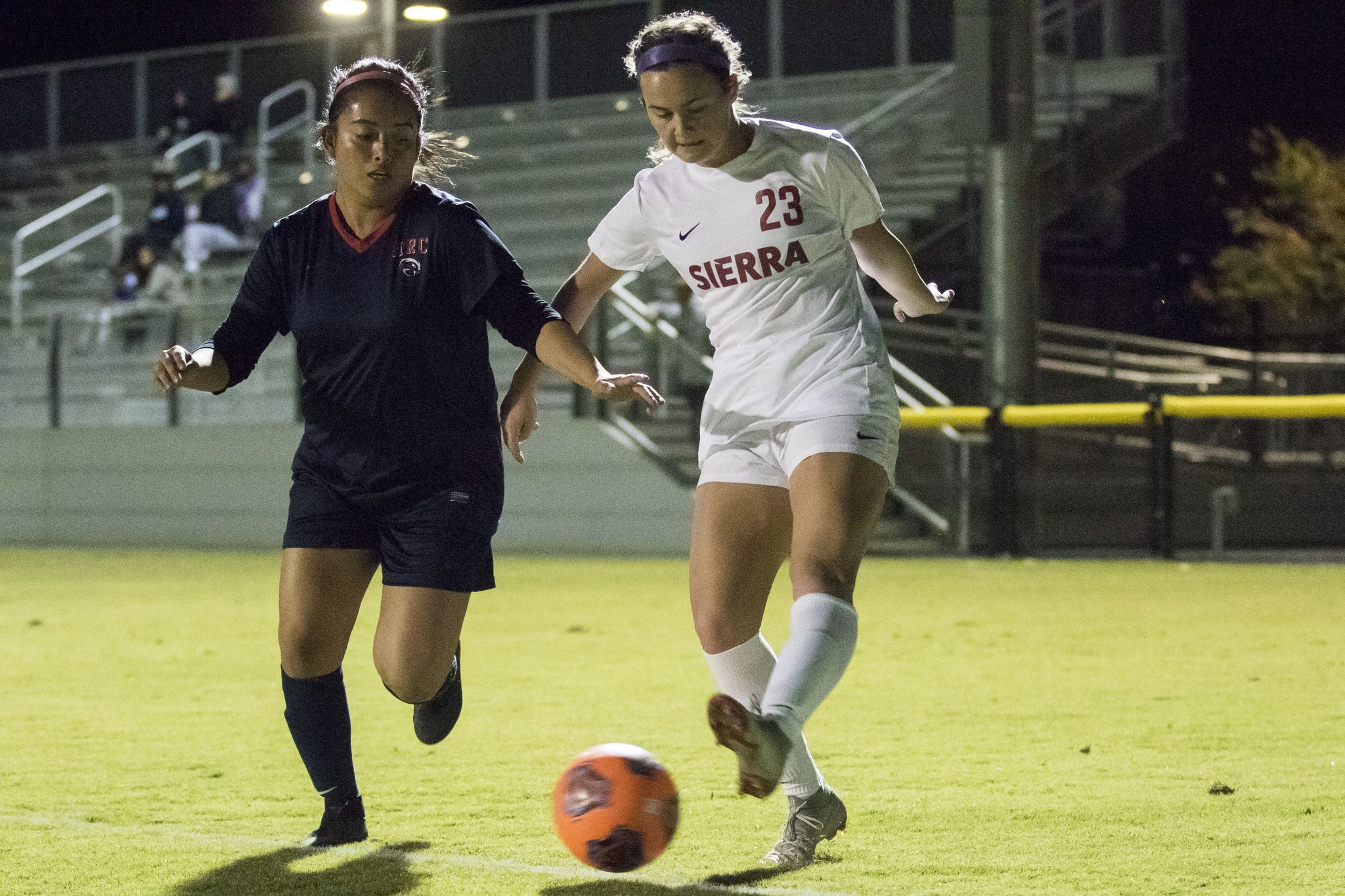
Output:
xmin=553 ymin=745 xmax=678 ymax=871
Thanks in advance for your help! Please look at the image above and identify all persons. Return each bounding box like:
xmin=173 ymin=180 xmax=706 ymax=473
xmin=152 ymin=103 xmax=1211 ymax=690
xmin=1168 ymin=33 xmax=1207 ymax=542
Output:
xmin=112 ymin=171 xmax=187 ymax=279
xmin=198 ymin=167 xmax=239 ymax=231
xmin=131 ymin=244 xmax=187 ymax=302
xmin=156 ymin=57 xmax=667 ymax=850
xmin=181 ymin=152 xmax=264 ymax=268
xmin=500 ymin=9 xmax=955 ymax=872
xmin=154 ymin=89 xmax=203 ymax=155
xmin=196 ymin=73 xmax=254 ymax=165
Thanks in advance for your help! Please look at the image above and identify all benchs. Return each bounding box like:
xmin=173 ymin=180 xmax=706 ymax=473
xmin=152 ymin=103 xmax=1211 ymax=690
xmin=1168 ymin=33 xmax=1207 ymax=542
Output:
xmin=0 ymin=63 xmax=956 ymax=342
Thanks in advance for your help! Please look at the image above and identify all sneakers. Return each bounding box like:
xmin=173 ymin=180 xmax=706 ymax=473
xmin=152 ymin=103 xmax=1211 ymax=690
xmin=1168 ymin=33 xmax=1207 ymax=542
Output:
xmin=306 ymin=794 xmax=367 ymax=849
xmin=707 ymin=690 xmax=793 ymax=798
xmin=762 ymin=784 xmax=847 ymax=870
xmin=412 ymin=641 xmax=463 ymax=746
